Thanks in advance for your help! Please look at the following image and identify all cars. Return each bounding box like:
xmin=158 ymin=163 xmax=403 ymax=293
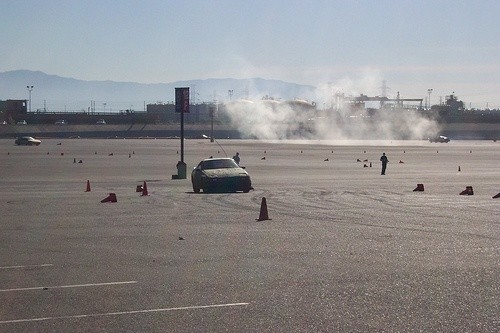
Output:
xmin=17 ymin=120 xmax=27 ymax=125
xmin=429 ymin=136 xmax=450 ymax=143
xmin=15 ymin=136 xmax=41 ymax=146
xmin=191 ymin=158 xmax=252 ymax=193
xmin=0 ymin=120 xmax=8 ymax=125
xmin=56 ymin=120 xmax=65 ymax=124
xmin=97 ymin=119 xmax=106 ymax=124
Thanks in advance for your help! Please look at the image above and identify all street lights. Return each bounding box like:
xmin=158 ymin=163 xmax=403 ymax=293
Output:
xmin=26 ymin=86 xmax=34 ymax=112
xmin=427 ymin=89 xmax=433 ymax=109
xmin=228 ymin=90 xmax=234 ymax=101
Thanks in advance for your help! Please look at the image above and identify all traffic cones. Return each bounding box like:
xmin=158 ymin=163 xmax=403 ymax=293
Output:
xmin=492 ymin=192 xmax=500 ymax=198
xmin=84 ymin=180 xmax=93 ymax=192
xmin=140 ymin=180 xmax=150 ymax=196
xmin=7 ymin=142 xmax=136 ymax=163
xmin=261 ymin=150 xmax=439 ymax=168
xmin=459 ymin=186 xmax=474 ymax=195
xmin=413 ymin=184 xmax=424 ymax=191
xmin=101 ymin=193 xmax=117 ymax=203
xmin=458 ymin=166 xmax=461 ymax=171
xmin=255 ymin=196 xmax=273 ymax=221
xmin=136 ymin=185 xmax=143 ymax=192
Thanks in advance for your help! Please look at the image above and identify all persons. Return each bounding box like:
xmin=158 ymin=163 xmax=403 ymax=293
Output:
xmin=380 ymin=153 xmax=388 ymax=175
xmin=233 ymin=152 xmax=240 ymax=163
